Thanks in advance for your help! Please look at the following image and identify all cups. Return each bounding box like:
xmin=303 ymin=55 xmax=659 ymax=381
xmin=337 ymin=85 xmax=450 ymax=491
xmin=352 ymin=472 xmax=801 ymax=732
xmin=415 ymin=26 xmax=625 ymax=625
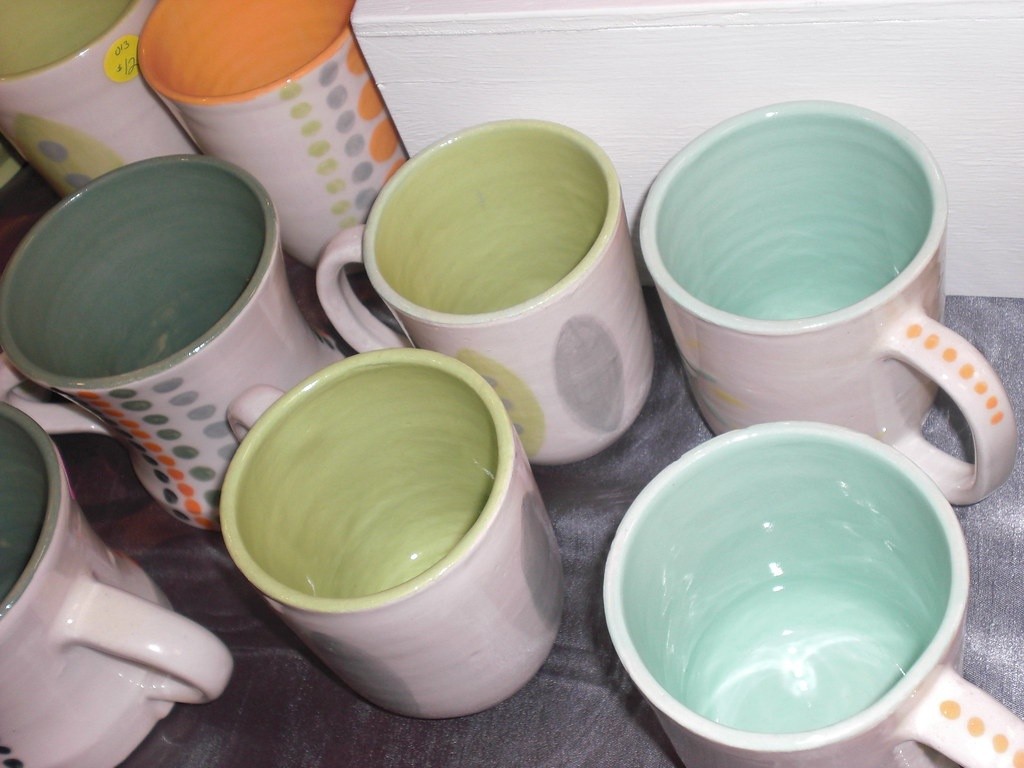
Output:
xmin=604 ymin=422 xmax=1024 ymax=768
xmin=0 ymin=154 xmax=350 ymax=530
xmin=315 ymin=120 xmax=655 ymax=463
xmin=0 ymin=401 xmax=233 ymax=768
xmin=137 ymin=0 xmax=409 ymax=268
xmin=220 ymin=349 xmax=567 ymax=719
xmin=639 ymin=101 xmax=1017 ymax=505
xmin=0 ymin=0 xmax=203 ymax=198
